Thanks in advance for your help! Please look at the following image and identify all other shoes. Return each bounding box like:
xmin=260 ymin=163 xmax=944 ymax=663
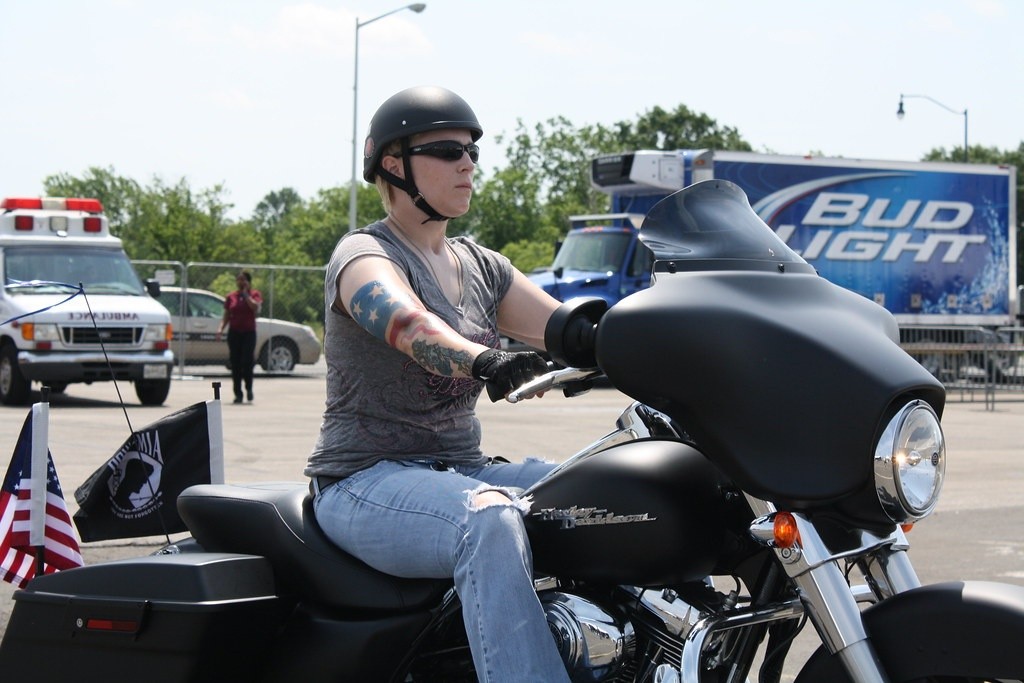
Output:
xmin=234 ymin=398 xmax=242 ymax=403
xmin=248 ymin=392 xmax=253 ymax=400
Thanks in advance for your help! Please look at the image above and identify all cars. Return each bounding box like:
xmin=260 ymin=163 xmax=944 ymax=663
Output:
xmin=144 ymin=284 xmax=322 ymax=378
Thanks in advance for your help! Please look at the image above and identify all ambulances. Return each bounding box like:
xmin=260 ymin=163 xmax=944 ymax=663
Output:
xmin=0 ymin=197 xmax=175 ymax=408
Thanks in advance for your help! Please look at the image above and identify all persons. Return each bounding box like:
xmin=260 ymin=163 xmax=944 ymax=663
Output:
xmin=84 ymin=266 xmax=100 ymax=282
xmin=302 ymin=85 xmax=575 ymax=683
xmin=218 ymin=271 xmax=263 ymax=403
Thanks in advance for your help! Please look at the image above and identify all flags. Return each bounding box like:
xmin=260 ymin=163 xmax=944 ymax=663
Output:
xmin=73 ymin=398 xmax=224 ymax=542
xmin=0 ymin=403 xmax=85 ymax=587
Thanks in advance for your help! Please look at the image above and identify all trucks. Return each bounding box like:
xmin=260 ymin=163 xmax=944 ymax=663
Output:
xmin=521 ymin=147 xmax=1020 ymax=383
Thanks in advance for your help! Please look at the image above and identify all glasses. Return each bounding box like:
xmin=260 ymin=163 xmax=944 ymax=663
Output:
xmin=391 ymin=140 xmax=479 ymax=164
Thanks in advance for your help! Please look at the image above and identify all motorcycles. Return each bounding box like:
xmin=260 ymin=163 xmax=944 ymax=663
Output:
xmin=1 ymin=179 xmax=1024 ymax=683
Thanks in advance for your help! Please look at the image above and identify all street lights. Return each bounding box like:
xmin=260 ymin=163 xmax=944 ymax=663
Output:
xmin=348 ymin=0 xmax=427 ymax=233
xmin=893 ymin=91 xmax=970 ymax=162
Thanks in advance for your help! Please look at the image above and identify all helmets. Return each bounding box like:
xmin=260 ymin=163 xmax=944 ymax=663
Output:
xmin=363 ymin=85 xmax=483 ymax=184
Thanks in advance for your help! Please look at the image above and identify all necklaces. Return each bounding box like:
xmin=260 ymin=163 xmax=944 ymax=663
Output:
xmin=388 ymin=214 xmax=461 ymax=305
